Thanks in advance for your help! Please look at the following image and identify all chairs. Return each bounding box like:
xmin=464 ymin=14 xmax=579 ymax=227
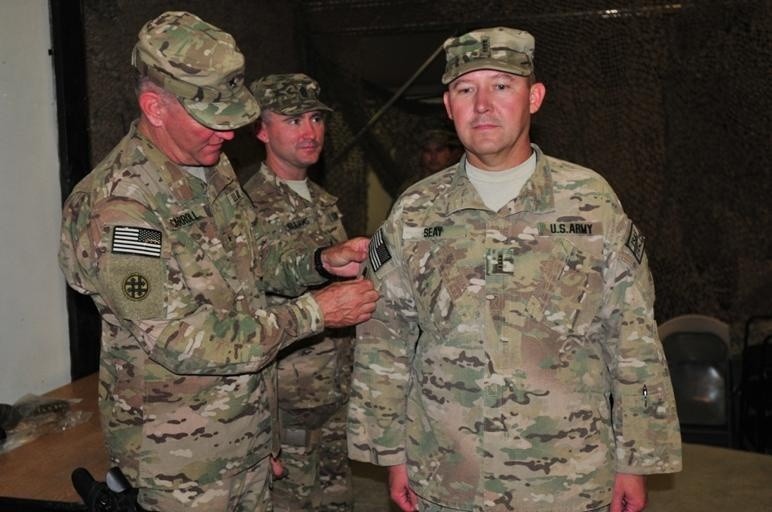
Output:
xmin=657 ymin=313 xmax=733 ymax=448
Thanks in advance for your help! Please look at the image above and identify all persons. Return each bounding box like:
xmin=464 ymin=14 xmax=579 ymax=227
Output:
xmin=384 ymin=130 xmax=459 ymax=221
xmin=242 ymin=72 xmax=354 ymax=511
xmin=60 ymin=13 xmax=379 ymax=510
xmin=344 ymin=27 xmax=683 ymax=512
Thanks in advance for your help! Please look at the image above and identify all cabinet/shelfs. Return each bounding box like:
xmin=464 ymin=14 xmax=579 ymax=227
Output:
xmin=737 ymin=314 xmax=772 ymax=455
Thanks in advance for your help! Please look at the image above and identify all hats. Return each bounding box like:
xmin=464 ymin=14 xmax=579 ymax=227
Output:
xmin=250 ymin=71 xmax=335 ymax=119
xmin=440 ymin=26 xmax=537 ymax=85
xmin=129 ymin=9 xmax=261 ymax=133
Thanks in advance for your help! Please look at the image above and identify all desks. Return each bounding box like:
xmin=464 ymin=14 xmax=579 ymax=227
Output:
xmin=0 ymin=371 xmax=113 ymax=504
xmin=641 ymin=443 xmax=772 ymax=512
